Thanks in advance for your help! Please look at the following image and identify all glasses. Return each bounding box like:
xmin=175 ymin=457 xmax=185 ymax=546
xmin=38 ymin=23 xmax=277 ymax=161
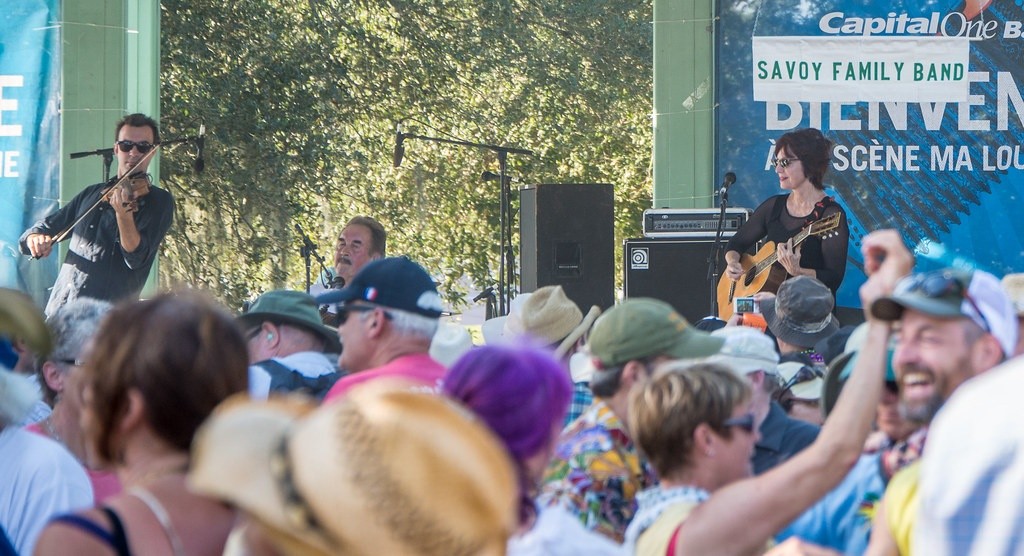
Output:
xmin=775 ymin=365 xmax=825 ymax=403
xmin=116 ymin=140 xmax=154 ymax=154
xmin=892 ymin=272 xmax=990 ymax=337
xmin=771 ymin=156 xmax=803 ymax=167
xmin=690 ymin=414 xmax=753 ymax=437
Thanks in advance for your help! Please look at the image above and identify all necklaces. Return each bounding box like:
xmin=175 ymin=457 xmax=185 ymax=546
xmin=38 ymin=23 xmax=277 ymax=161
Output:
xmin=45 ymin=415 xmax=88 ymax=465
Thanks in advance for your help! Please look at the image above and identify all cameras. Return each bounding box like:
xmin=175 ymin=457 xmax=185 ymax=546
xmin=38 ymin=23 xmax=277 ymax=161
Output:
xmin=734 ymin=297 xmax=761 ymax=315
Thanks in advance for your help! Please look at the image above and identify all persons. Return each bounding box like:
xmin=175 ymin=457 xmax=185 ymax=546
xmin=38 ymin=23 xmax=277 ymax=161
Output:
xmin=726 ymin=128 xmax=850 ymax=317
xmin=0 ymin=227 xmax=1023 ymax=556
xmin=20 ymin=113 xmax=174 ymax=319
xmin=315 ymin=216 xmax=386 ymax=287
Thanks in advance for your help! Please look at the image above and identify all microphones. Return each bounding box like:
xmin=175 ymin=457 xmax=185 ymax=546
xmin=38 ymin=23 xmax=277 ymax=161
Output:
xmin=193 ymin=121 xmax=205 ymax=172
xmin=393 ymin=122 xmax=404 ymax=167
xmin=333 ymin=276 xmax=345 ymax=290
xmin=482 ymin=172 xmax=522 ymax=183
xmin=720 ymin=172 xmax=736 ymax=195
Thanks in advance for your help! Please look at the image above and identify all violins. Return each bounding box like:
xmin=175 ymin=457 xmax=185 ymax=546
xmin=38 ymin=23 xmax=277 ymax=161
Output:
xmin=98 ymin=172 xmax=151 ymax=213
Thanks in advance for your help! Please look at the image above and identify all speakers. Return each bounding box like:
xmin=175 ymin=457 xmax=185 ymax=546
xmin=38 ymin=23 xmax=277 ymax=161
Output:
xmin=520 ymin=183 xmax=614 ymax=322
xmin=624 ymin=237 xmax=731 ymax=327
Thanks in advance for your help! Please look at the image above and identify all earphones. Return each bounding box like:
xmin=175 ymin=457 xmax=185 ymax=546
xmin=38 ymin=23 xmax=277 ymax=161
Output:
xmin=267 ymin=332 xmax=273 ymax=341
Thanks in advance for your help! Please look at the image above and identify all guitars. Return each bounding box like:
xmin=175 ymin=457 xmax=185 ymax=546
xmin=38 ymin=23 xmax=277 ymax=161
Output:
xmin=716 ymin=210 xmax=843 ymax=323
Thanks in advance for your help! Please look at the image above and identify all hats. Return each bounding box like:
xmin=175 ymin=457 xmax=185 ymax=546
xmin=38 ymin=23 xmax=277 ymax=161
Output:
xmin=696 ymin=326 xmax=779 ymax=377
xmin=589 ymin=298 xmax=724 ymax=367
xmin=186 ymin=374 xmax=518 ymax=556
xmin=445 ymin=345 xmax=571 ymax=457
xmin=522 ymin=285 xmax=582 ymax=350
xmin=871 ymin=268 xmax=1017 ymax=359
xmin=777 ymin=361 xmax=825 ymax=401
xmin=759 ymin=276 xmax=839 ymax=348
xmin=820 ymin=321 xmax=902 ymax=420
xmin=429 ymin=324 xmax=474 ymax=367
xmin=480 ymin=293 xmax=533 ymax=345
xmin=315 ymin=255 xmax=443 ymax=317
xmin=230 ymin=289 xmax=342 ymax=355
